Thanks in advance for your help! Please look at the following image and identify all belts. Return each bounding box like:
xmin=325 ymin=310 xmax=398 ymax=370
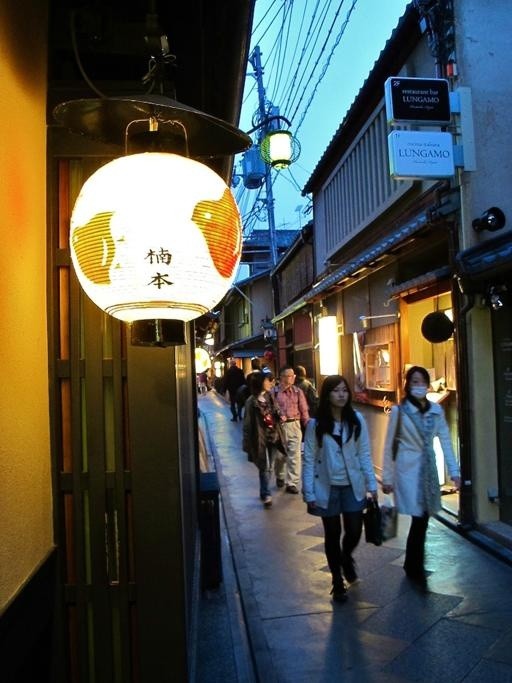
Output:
xmin=281 ymin=419 xmax=300 ymax=422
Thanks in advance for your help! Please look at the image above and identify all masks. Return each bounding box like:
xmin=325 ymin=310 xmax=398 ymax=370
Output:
xmin=411 ymin=386 xmax=429 ymax=398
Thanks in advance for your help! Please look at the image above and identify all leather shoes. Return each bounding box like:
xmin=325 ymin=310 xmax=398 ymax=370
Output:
xmin=231 ymin=417 xmax=243 ymax=422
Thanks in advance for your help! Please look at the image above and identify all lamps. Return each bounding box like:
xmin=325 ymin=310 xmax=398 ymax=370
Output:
xmin=247 ymin=113 xmax=303 ymax=170
xmin=49 ymin=35 xmax=252 ymax=346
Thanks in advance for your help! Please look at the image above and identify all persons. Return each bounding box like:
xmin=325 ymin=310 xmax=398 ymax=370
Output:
xmin=242 ymin=371 xmax=289 ymax=505
xmin=273 ymin=367 xmax=309 ymax=495
xmin=382 ymin=364 xmax=466 ymax=594
xmin=302 ymin=373 xmax=381 ymax=603
xmin=198 ymin=358 xmax=318 ymax=421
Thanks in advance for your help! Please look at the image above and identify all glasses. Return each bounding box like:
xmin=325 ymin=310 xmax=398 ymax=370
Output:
xmin=281 ymin=375 xmax=296 ymax=378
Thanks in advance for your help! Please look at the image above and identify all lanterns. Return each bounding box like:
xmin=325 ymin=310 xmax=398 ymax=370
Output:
xmin=68 ymin=150 xmax=244 ymax=349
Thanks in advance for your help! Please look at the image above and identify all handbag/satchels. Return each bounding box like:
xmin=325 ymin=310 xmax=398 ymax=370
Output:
xmin=381 ymin=494 xmax=398 ymax=542
xmin=363 ymin=495 xmax=384 ymax=546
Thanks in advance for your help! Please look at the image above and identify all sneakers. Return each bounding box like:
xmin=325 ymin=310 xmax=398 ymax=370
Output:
xmin=402 ymin=564 xmax=430 ymax=595
xmin=264 ymin=496 xmax=272 ymax=507
xmin=341 ymin=553 xmax=357 ymax=583
xmin=276 ymin=478 xmax=284 ymax=488
xmin=330 ymin=578 xmax=346 ymax=601
xmin=286 ymin=487 xmax=298 ymax=495
